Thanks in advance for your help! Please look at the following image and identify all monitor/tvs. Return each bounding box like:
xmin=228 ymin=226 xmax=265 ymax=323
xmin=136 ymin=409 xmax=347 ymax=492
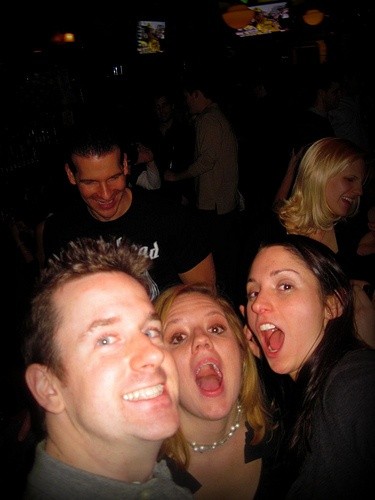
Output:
xmin=137 ymin=21 xmax=167 ymax=54
xmin=234 ymin=0 xmax=292 ymax=38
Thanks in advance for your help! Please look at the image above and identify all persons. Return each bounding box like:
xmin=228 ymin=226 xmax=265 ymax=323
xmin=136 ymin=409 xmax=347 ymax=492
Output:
xmin=256 ymin=136 xmax=375 ymax=351
xmin=133 ymin=144 xmax=162 ymax=191
xmin=163 ymin=80 xmax=239 ymax=303
xmin=151 ymin=90 xmax=195 ymax=214
xmin=20 ymin=235 xmax=201 ymax=500
xmin=40 ymin=127 xmax=218 ymax=296
xmin=249 ymin=63 xmax=375 ymax=153
xmin=150 ymin=281 xmax=296 ymax=500
xmin=238 ymin=232 xmax=375 ymax=500
xmin=0 ymin=216 xmax=55 ymax=499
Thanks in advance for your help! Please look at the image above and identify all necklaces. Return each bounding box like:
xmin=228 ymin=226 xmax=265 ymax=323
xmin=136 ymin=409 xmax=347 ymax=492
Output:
xmin=184 ymin=404 xmax=244 ymax=451
